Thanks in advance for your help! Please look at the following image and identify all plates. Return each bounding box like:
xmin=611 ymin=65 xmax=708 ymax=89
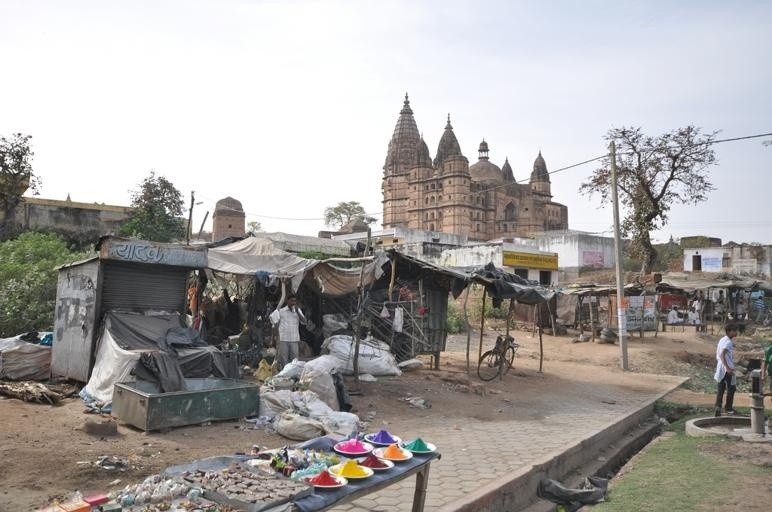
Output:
xmin=328 ymin=464 xmax=375 ymax=480
xmin=372 ymin=446 xmax=414 ymax=462
xmin=364 ymin=432 xmax=402 ymax=446
xmin=333 ymin=440 xmax=374 ymax=455
xmin=398 ymin=440 xmax=437 ymax=454
xmin=353 ymin=457 xmax=395 ymax=471
xmin=300 ymin=474 xmax=349 ymax=491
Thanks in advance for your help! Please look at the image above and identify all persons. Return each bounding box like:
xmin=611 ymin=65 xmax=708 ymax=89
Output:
xmin=667 ymin=305 xmax=685 ymax=325
xmin=713 ymin=322 xmax=739 ymax=418
xmin=687 ymin=289 xmax=766 ymax=332
xmin=759 ymin=344 xmax=772 ymax=400
xmin=269 ymin=292 xmax=307 ymax=376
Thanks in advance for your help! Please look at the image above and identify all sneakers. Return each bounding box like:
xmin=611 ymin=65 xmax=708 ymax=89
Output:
xmin=727 ymin=409 xmax=739 ymax=416
xmin=714 ymin=406 xmax=721 ymax=417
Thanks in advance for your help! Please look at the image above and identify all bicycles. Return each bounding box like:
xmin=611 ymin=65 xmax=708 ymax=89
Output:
xmin=476 ymin=324 xmax=519 ymax=382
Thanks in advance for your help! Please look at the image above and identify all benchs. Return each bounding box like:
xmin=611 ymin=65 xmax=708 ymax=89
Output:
xmin=661 ymin=321 xmax=708 ymax=332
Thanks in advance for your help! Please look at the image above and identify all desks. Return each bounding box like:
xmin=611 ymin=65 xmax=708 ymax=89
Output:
xmin=38 ymin=429 xmax=440 ymax=512
